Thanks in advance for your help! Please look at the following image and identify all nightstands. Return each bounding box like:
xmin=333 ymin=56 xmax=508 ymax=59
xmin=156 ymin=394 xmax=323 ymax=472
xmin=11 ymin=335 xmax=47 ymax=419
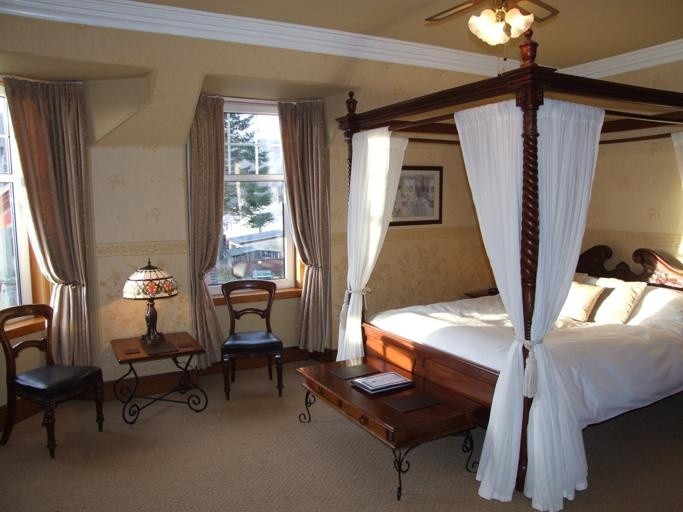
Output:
xmin=462 ymin=291 xmax=493 ymax=298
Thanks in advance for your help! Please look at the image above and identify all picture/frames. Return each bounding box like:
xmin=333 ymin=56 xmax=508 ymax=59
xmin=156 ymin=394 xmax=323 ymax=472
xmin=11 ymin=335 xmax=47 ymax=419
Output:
xmin=389 ymin=161 xmax=444 ymax=227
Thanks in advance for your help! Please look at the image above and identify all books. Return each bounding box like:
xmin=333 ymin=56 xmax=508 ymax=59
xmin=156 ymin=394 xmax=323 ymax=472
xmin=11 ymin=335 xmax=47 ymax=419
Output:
xmin=351 ymin=371 xmax=416 ymax=397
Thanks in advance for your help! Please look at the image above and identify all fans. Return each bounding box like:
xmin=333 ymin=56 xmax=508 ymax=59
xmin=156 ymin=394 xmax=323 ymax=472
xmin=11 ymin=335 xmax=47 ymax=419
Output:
xmin=424 ymin=0 xmax=566 ymax=25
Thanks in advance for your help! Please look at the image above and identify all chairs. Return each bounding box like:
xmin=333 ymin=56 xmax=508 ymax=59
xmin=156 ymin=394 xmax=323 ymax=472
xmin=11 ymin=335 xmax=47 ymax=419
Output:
xmin=221 ymin=279 xmax=285 ymax=400
xmin=1 ymin=299 xmax=104 ymax=461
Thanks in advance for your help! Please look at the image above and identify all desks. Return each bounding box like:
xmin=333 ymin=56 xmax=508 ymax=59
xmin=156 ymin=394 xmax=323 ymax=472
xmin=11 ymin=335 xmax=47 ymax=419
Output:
xmin=297 ymin=350 xmax=494 ymax=501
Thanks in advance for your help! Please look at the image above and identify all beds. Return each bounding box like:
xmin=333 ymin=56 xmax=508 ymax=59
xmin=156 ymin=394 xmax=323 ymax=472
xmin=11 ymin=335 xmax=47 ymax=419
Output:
xmin=335 ymin=27 xmax=683 ymax=489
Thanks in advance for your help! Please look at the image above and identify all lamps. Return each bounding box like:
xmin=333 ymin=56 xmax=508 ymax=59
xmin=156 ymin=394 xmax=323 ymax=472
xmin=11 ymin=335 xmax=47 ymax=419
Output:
xmin=469 ymin=2 xmax=536 ymax=47
xmin=123 ymin=259 xmax=178 ymax=345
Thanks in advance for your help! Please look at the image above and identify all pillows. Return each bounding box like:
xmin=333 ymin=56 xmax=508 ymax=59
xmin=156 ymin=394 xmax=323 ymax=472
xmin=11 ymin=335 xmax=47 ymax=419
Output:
xmin=558 ymin=273 xmax=647 ymax=326
